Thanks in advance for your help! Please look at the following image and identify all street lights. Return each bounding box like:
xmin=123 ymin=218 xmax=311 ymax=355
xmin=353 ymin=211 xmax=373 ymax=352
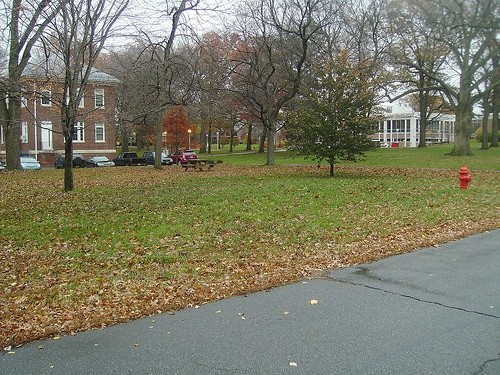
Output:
xmin=188 ymin=129 xmax=192 ymax=150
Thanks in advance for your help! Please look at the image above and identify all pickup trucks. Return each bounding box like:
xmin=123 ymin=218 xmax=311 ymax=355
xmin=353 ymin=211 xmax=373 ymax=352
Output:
xmin=112 ymin=152 xmax=147 ymax=166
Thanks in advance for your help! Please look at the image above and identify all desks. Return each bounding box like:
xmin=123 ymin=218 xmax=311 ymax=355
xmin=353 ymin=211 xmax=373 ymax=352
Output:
xmin=185 ymin=159 xmax=208 ymax=172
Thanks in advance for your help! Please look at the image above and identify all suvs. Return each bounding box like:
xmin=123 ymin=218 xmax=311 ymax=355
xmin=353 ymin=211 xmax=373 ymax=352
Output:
xmin=168 ymin=149 xmax=198 ymax=165
xmin=142 ymin=151 xmax=173 ymax=165
xmin=54 ymin=154 xmax=86 ymax=169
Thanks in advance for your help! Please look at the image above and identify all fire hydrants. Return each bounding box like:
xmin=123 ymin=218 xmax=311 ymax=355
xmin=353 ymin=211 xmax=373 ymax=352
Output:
xmin=458 ymin=166 xmax=472 ymax=190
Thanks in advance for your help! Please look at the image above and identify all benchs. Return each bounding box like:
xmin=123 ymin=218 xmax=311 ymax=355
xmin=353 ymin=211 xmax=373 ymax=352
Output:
xmin=182 ymin=164 xmax=214 ymax=172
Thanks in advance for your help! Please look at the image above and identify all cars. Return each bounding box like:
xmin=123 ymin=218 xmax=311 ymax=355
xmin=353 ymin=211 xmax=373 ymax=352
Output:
xmin=18 ymin=156 xmax=41 ymax=170
xmin=84 ymin=155 xmax=115 ymax=167
